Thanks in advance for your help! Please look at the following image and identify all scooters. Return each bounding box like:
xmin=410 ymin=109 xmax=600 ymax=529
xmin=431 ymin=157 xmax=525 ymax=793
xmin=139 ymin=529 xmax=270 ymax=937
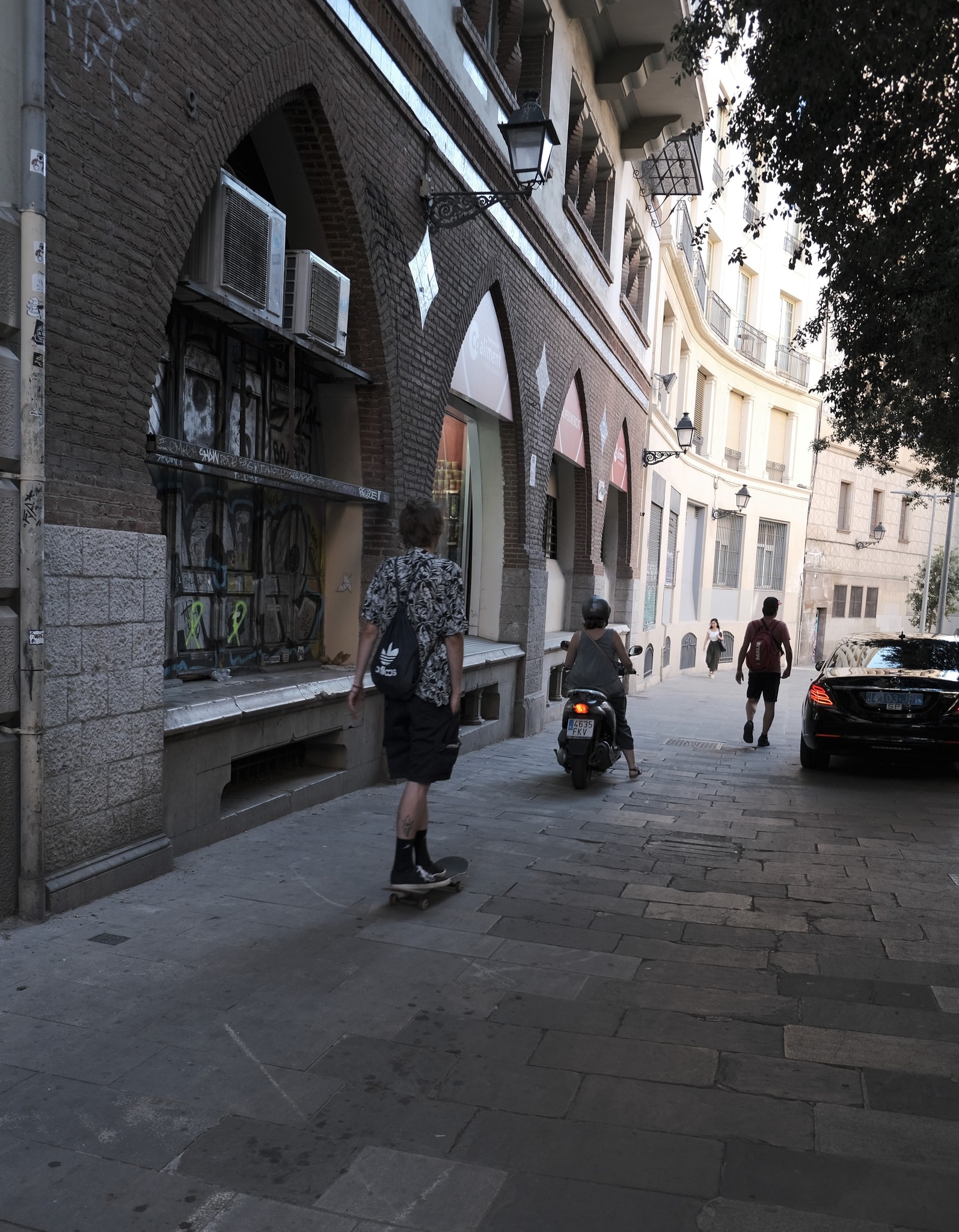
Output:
xmin=553 ymin=640 xmax=643 ymax=790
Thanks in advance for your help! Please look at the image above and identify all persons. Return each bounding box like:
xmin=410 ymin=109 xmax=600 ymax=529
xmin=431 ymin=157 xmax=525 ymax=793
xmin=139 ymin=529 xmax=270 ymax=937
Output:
xmin=565 ymin=594 xmax=643 ymax=779
xmin=736 ymin=597 xmax=793 ymax=747
xmin=348 ymin=497 xmax=470 ymax=889
xmin=703 ymin=618 xmax=724 ymax=679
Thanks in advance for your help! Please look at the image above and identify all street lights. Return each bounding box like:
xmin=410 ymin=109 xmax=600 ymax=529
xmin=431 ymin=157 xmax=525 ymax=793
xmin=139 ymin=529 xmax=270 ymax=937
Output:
xmin=890 ymin=491 xmax=959 ymax=632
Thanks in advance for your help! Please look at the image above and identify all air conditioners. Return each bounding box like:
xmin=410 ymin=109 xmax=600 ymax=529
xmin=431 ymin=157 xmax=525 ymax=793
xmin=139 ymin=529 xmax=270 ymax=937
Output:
xmin=283 ymin=249 xmax=350 ymax=358
xmin=180 ymin=168 xmax=286 ymax=328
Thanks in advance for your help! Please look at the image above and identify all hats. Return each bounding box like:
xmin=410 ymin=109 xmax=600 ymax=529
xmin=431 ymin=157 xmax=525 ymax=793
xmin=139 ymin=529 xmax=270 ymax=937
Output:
xmin=763 ymin=597 xmax=782 ymax=607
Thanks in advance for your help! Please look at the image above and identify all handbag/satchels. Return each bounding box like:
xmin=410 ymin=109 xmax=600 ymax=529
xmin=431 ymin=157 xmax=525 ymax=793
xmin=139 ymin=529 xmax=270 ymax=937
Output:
xmin=718 ymin=629 xmax=726 ymax=652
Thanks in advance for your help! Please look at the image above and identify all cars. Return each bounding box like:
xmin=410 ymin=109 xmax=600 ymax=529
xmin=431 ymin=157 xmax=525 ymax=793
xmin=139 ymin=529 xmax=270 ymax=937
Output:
xmin=800 ymin=631 xmax=959 ymax=772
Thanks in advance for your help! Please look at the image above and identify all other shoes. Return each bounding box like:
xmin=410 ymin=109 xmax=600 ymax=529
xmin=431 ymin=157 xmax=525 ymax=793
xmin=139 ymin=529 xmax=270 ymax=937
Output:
xmin=758 ymin=736 xmax=770 ymax=746
xmin=743 ymin=721 xmax=754 ymax=743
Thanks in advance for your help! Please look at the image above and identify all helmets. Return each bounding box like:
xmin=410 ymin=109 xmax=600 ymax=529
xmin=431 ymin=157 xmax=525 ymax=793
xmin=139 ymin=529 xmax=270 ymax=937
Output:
xmin=581 ymin=595 xmax=611 ymax=621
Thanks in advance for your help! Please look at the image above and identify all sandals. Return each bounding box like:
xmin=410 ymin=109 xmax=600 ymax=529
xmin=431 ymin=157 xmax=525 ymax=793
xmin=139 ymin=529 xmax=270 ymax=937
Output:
xmin=709 ymin=671 xmax=712 ymax=677
xmin=710 ymin=675 xmax=714 ymax=678
xmin=629 ymin=766 xmax=642 ymax=779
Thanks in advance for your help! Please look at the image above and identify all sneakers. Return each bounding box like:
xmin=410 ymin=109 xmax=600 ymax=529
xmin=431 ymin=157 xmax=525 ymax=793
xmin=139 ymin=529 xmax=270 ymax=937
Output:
xmin=391 ymin=865 xmax=451 ymax=889
xmin=422 ymin=863 xmax=446 ymax=877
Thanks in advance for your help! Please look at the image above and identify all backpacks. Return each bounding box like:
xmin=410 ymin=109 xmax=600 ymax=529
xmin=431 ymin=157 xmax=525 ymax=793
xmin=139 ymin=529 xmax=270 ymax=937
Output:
xmin=746 ymin=619 xmax=785 ymax=671
xmin=370 ymin=554 xmax=443 ymax=702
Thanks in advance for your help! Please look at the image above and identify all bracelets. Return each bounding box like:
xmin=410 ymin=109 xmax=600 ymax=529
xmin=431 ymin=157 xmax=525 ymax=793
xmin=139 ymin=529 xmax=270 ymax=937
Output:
xmin=350 ymin=685 xmax=365 ymax=694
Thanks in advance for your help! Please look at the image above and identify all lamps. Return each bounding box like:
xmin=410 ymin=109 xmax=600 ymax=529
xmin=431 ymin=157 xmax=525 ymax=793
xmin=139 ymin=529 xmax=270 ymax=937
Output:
xmin=642 ymin=411 xmax=697 ymax=468
xmin=711 ymin=484 xmax=752 ymax=520
xmin=654 ymin=373 xmax=677 ymax=394
xmin=423 ymin=90 xmax=561 ymax=234
xmin=855 ymin=522 xmax=885 ymax=550
